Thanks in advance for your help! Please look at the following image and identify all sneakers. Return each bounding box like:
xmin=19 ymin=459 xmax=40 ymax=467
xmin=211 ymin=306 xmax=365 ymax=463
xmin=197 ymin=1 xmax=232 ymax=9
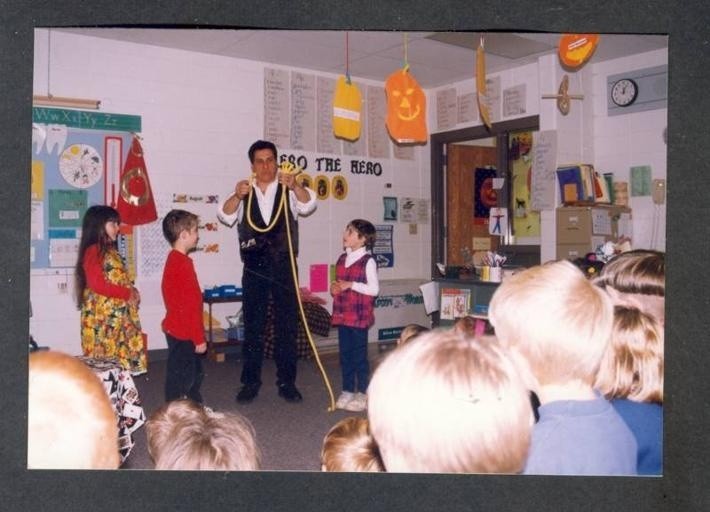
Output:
xmin=279 ymin=384 xmax=300 ymax=401
xmin=335 ymin=392 xmax=367 ymax=412
xmin=238 ymin=382 xmax=261 ymax=402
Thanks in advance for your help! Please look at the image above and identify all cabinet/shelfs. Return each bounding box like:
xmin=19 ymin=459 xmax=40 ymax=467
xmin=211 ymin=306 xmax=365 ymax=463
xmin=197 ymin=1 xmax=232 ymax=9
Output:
xmin=555 ymin=201 xmax=635 ymax=273
xmin=434 ymin=280 xmax=512 ymax=340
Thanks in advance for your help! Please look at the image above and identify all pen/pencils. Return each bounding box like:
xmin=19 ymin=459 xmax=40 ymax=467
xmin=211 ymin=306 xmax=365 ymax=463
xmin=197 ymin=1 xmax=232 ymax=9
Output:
xmin=480 ymin=251 xmax=507 ymax=266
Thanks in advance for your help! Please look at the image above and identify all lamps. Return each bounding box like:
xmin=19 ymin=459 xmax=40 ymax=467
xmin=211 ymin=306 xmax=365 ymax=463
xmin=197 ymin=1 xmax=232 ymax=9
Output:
xmin=33 ymin=28 xmax=103 ymax=110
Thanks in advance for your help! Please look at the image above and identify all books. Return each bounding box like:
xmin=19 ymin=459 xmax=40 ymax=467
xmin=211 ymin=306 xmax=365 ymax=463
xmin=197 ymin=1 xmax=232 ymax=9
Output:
xmin=557 ymin=162 xmax=615 ymax=205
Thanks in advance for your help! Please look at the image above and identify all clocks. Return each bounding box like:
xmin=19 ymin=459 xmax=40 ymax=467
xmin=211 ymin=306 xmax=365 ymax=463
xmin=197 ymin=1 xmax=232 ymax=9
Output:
xmin=612 ymin=78 xmax=640 ymax=108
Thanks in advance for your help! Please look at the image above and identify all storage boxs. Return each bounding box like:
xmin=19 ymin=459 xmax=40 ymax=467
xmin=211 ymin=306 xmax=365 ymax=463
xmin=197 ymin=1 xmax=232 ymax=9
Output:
xmin=370 ymin=280 xmax=435 ymax=346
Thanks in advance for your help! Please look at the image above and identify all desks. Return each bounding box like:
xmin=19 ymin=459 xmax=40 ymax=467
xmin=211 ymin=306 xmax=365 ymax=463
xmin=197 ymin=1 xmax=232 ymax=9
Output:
xmin=202 ymin=293 xmax=247 ymax=367
xmin=71 ymin=354 xmax=147 ymax=469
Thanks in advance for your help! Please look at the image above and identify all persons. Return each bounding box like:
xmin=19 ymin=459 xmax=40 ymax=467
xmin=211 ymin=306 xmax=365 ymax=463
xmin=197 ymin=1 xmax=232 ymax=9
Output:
xmin=215 ymin=139 xmax=317 ymax=402
xmin=160 ymin=208 xmax=208 ymax=403
xmin=591 ymin=249 xmax=664 ymax=475
xmin=28 ymin=350 xmax=122 ymax=469
xmin=368 ymin=327 xmax=535 ymax=474
xmin=330 ymin=218 xmax=381 ymax=410
xmin=318 ymin=416 xmax=389 ymax=471
xmin=144 ymin=397 xmax=266 ymax=470
xmin=73 ymin=205 xmax=148 ymax=469
xmin=488 ymin=258 xmax=639 ymax=473
xmin=398 ymin=325 xmax=429 ymax=345
